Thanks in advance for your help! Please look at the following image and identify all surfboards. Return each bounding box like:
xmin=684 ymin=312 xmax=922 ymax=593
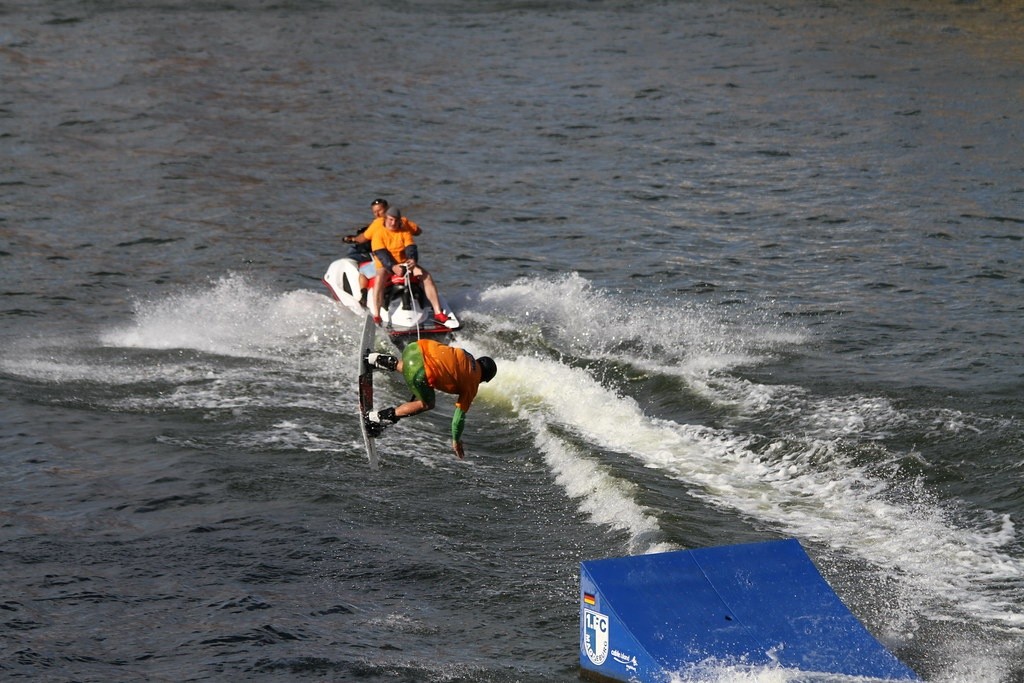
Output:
xmin=358 ymin=315 xmax=377 ymax=459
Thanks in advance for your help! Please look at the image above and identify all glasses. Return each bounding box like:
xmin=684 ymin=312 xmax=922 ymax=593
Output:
xmin=372 ymin=200 xmax=382 ymax=204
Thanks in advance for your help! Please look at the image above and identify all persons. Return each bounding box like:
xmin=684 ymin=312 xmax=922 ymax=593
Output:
xmin=344 ymin=199 xmax=423 ymax=307
xmin=371 ymin=206 xmax=449 ymax=325
xmin=363 ymin=339 xmax=497 ymax=458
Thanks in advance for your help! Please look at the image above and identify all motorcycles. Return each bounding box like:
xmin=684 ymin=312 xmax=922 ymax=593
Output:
xmin=324 ymin=227 xmax=459 ymax=334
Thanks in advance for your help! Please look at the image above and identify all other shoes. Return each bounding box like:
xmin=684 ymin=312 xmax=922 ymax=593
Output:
xmin=373 ymin=315 xmax=384 ymax=326
xmin=433 ymin=312 xmax=449 ymax=323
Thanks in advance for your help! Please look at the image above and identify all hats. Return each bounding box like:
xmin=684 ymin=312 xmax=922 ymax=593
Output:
xmin=387 ymin=206 xmax=401 ymax=218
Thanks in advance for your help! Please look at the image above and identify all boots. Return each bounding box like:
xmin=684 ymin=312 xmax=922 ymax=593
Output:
xmin=362 ymin=347 xmax=398 ymax=373
xmin=364 ymin=407 xmax=401 ymax=437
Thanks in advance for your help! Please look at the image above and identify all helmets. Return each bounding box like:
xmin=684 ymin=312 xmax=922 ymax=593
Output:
xmin=478 ymin=356 xmax=497 ymax=383
